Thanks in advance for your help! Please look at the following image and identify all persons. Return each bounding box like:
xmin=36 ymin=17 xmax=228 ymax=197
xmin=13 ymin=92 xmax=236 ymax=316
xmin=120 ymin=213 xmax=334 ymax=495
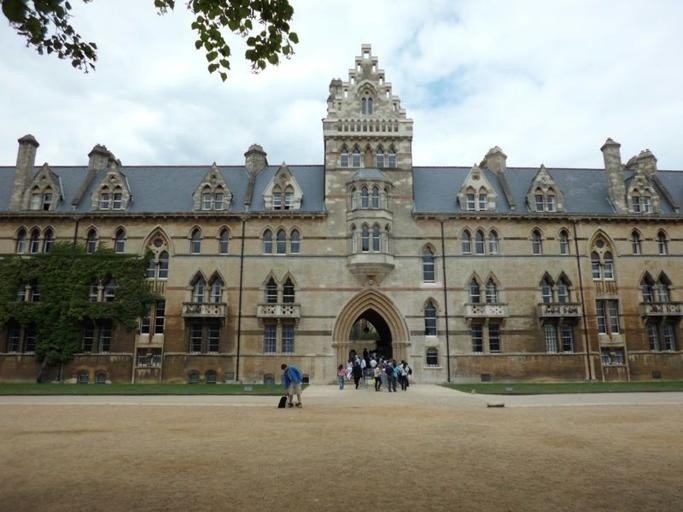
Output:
xmin=336 ymin=364 xmax=346 ymax=390
xmin=344 ymin=347 xmax=412 ymax=392
xmin=280 ymin=363 xmax=304 ymax=408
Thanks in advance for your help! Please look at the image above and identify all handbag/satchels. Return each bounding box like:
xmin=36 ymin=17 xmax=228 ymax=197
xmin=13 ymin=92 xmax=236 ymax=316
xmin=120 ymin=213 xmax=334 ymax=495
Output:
xmin=278 ymin=397 xmax=287 ymax=408
